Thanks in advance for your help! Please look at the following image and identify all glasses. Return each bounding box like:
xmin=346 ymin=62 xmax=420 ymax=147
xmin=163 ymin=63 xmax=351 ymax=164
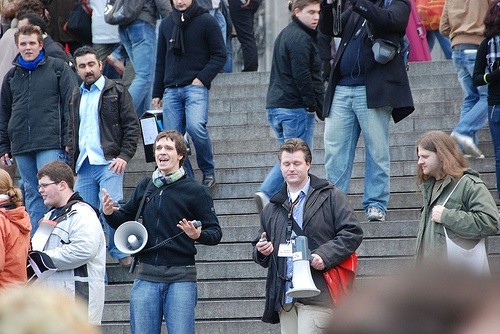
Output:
xmin=37 ymin=182 xmax=58 ymax=188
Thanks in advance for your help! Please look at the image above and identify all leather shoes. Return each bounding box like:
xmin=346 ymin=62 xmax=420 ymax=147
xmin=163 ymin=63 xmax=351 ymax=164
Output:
xmin=202 ymin=172 xmax=216 ymax=190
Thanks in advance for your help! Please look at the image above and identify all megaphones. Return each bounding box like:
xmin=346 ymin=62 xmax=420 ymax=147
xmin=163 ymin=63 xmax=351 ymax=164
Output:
xmin=287 ymin=236 xmax=321 ymax=298
xmin=113 ymin=220 xmax=149 ymax=254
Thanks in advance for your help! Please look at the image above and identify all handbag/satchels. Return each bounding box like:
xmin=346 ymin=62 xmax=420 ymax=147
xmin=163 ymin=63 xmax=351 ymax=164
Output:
xmin=69 ymin=0 xmax=93 ymax=39
xmin=104 ymin=0 xmax=145 ymax=25
xmin=58 ymin=16 xmax=77 ymax=42
xmin=323 ymin=252 xmax=358 ymax=308
xmin=26 ymin=249 xmax=58 ymax=288
xmin=384 ymin=0 xmax=411 ymax=71
xmin=441 ymin=174 xmax=491 ymax=280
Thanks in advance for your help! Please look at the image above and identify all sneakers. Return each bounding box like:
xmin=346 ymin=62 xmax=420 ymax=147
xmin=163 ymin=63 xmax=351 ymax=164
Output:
xmin=365 ymin=207 xmax=385 ymax=221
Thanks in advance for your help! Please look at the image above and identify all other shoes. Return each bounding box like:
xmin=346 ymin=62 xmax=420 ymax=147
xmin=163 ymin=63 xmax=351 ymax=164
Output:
xmin=241 ymin=69 xmax=256 ymax=72
xmin=107 ymin=54 xmax=125 ymax=75
xmin=118 ymin=255 xmax=132 ymax=268
xmin=255 ymin=193 xmax=270 ymax=214
xmin=451 ymin=131 xmax=485 ymax=159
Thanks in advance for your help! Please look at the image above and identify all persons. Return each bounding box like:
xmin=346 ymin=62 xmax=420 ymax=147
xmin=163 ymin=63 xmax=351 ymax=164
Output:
xmin=251 ymin=137 xmax=364 ymax=334
xmin=0 ymin=0 xmax=500 ymax=334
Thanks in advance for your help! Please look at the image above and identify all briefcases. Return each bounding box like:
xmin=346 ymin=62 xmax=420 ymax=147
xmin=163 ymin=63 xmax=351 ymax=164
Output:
xmin=139 ymin=109 xmax=163 ymax=163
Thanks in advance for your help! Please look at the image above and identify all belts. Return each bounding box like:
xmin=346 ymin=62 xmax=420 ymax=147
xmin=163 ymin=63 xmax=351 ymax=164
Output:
xmin=452 ymin=45 xmax=478 ymax=50
